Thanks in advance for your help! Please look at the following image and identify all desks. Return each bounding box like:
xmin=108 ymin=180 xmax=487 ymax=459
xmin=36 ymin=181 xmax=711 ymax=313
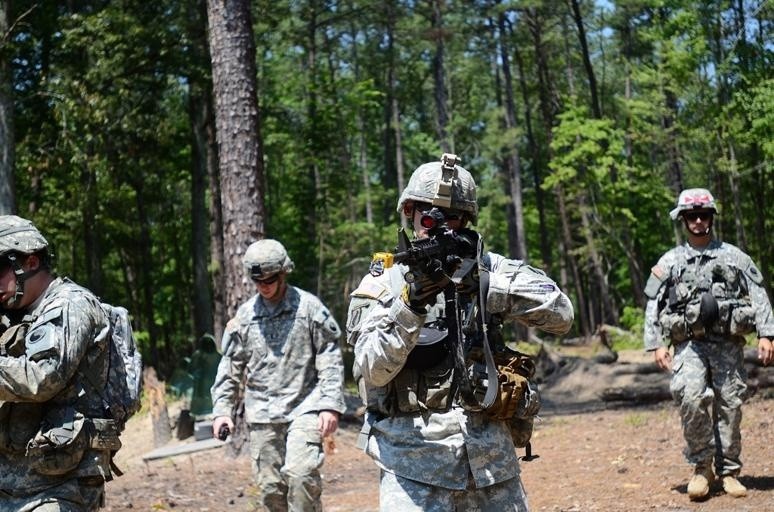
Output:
xmin=143 ymin=433 xmax=234 ymax=480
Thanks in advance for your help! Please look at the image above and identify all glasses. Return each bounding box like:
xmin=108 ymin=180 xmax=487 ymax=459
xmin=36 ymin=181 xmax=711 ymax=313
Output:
xmin=681 ymin=210 xmax=713 ymax=222
xmin=249 ymin=273 xmax=281 ymax=286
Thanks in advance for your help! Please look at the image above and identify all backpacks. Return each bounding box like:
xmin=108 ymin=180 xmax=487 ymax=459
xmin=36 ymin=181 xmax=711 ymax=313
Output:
xmin=102 ymin=300 xmax=145 ymax=423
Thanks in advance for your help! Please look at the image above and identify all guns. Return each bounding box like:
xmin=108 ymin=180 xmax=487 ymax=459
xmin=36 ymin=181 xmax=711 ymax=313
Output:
xmin=370 ymin=212 xmax=493 ymax=338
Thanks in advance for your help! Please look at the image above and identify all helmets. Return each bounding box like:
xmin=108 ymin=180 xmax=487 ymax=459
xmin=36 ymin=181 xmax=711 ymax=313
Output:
xmin=395 ymin=153 xmax=478 ymax=226
xmin=239 ymin=239 xmax=297 ymax=281
xmin=0 ymin=213 xmax=51 ymax=267
xmin=668 ymin=186 xmax=719 ymax=222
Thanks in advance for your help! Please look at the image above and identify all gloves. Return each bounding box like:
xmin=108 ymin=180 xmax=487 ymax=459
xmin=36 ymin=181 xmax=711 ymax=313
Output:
xmin=402 ymin=274 xmax=441 ymax=313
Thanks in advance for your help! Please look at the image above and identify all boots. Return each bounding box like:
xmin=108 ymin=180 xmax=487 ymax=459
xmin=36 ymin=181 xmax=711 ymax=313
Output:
xmin=718 ymin=469 xmax=748 ymax=498
xmin=686 ymin=458 xmax=716 ymax=499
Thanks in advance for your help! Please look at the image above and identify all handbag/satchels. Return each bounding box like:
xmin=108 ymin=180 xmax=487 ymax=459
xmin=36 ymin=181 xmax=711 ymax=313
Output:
xmin=467 ymin=342 xmax=537 ymax=460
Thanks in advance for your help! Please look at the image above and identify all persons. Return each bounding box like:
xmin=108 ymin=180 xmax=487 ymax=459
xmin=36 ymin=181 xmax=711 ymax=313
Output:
xmin=643 ymin=187 xmax=774 ymax=500
xmin=0 ymin=214 xmax=143 ymax=512
xmin=346 ymin=153 xmax=575 ymax=512
xmin=210 ymin=239 xmax=347 ymax=512
